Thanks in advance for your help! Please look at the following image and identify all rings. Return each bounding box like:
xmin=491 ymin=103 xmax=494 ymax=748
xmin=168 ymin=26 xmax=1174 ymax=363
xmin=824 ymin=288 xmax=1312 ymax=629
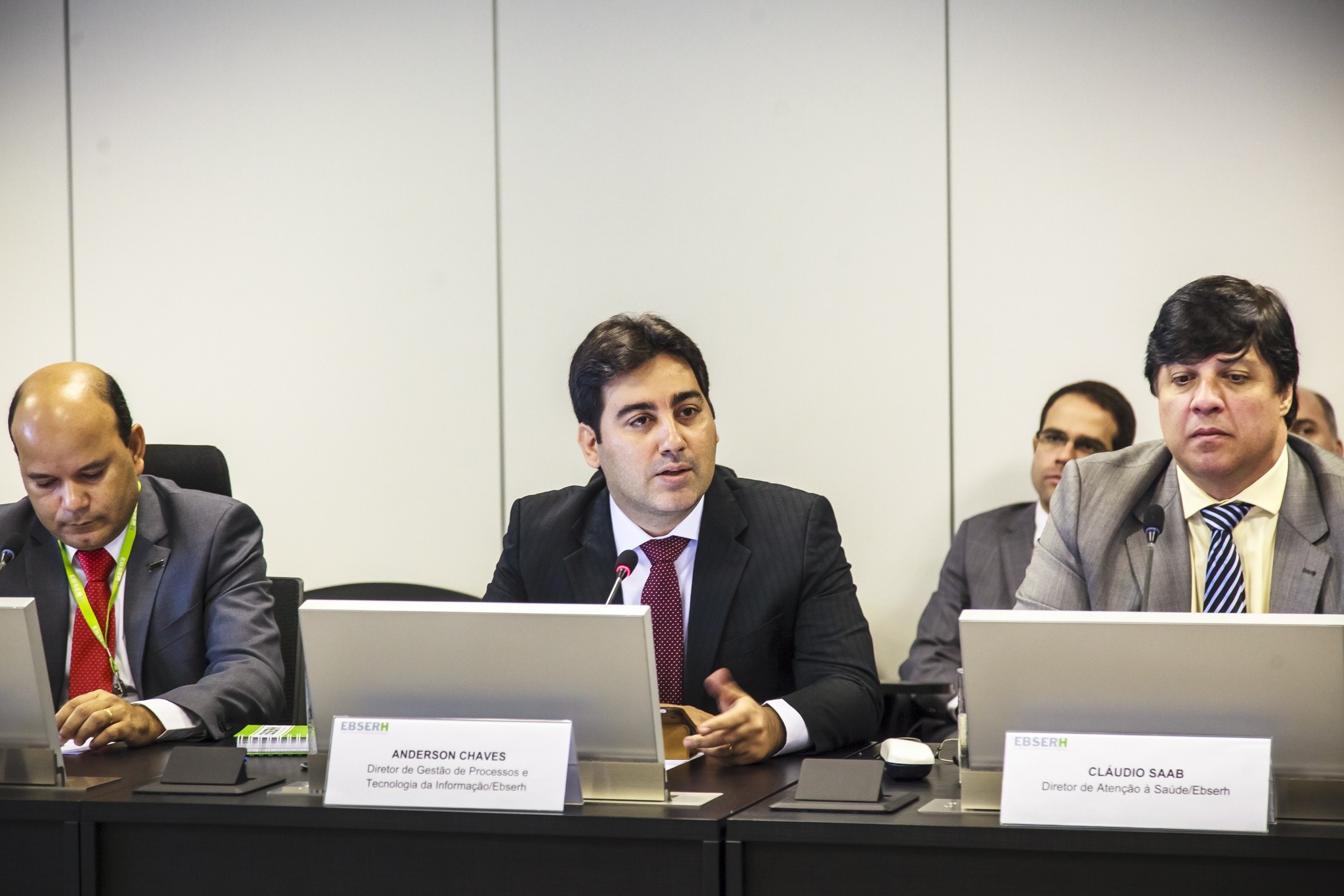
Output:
xmin=727 ymin=743 xmax=733 ymax=755
xmin=103 ymin=709 xmax=114 ymax=723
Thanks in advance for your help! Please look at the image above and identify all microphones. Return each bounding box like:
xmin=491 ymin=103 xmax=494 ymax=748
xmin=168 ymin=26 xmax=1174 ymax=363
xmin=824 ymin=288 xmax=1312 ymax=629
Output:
xmin=0 ymin=534 xmax=26 ymax=573
xmin=605 ymin=549 xmax=640 ymax=604
xmin=1141 ymin=503 xmax=1168 ymax=611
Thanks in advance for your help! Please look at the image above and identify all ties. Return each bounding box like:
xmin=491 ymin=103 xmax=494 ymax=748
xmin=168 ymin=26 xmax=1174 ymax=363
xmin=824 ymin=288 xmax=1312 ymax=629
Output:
xmin=68 ymin=548 xmax=116 ymax=700
xmin=639 ymin=536 xmax=685 ymax=705
xmin=1199 ymin=502 xmax=1256 ymax=613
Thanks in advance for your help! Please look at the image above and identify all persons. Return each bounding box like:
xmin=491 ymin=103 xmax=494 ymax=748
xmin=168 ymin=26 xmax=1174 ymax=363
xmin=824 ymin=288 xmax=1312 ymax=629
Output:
xmin=1288 ymin=387 xmax=1344 ymax=461
xmin=0 ymin=361 xmax=285 ymax=751
xmin=898 ymin=381 xmax=1136 ymax=743
xmin=1010 ymin=274 xmax=1344 ymax=614
xmin=481 ymin=311 xmax=883 ymax=766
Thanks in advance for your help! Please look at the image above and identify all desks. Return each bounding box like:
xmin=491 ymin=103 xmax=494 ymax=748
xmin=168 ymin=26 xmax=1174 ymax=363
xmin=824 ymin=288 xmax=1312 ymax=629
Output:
xmin=0 ymin=742 xmax=1344 ymax=896
